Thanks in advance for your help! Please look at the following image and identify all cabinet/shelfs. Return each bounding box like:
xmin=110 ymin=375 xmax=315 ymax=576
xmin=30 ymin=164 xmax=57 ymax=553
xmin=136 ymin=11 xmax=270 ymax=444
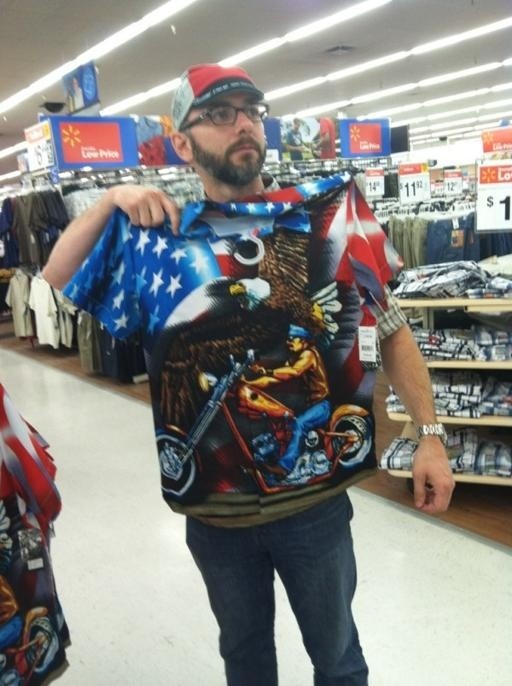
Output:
xmin=385 ymin=297 xmax=511 ymax=494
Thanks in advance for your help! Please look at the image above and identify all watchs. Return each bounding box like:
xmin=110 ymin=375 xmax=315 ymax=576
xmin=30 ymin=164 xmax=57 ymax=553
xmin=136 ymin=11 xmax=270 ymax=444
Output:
xmin=417 ymin=424 xmax=448 ymax=445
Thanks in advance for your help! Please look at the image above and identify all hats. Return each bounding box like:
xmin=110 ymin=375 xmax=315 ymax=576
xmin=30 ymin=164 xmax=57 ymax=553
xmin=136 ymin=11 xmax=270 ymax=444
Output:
xmin=288 ymin=324 xmax=312 ymax=338
xmin=171 ymin=64 xmax=265 ymax=132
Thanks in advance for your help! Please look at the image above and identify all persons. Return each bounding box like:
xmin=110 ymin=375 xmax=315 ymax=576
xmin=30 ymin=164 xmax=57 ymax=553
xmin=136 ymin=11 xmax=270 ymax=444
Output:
xmin=240 ymin=324 xmax=330 ymax=475
xmin=42 ymin=65 xmax=455 ymax=686
xmin=73 ymin=79 xmax=84 ymax=109
xmin=281 ymin=115 xmax=335 ymax=161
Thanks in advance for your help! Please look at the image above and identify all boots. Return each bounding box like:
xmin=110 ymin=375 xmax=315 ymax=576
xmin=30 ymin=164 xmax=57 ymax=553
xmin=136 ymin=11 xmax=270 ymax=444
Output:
xmin=263 ymin=462 xmax=290 ymax=478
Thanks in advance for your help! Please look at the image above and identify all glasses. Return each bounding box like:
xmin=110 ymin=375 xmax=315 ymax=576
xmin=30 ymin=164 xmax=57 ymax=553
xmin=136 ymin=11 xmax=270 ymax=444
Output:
xmin=288 ymin=336 xmax=296 ymax=341
xmin=180 ymin=102 xmax=270 ymax=130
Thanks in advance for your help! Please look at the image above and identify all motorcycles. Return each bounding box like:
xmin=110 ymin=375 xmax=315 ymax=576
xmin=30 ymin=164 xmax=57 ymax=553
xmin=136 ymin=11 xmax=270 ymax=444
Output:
xmin=156 ymin=348 xmax=376 ymax=500
